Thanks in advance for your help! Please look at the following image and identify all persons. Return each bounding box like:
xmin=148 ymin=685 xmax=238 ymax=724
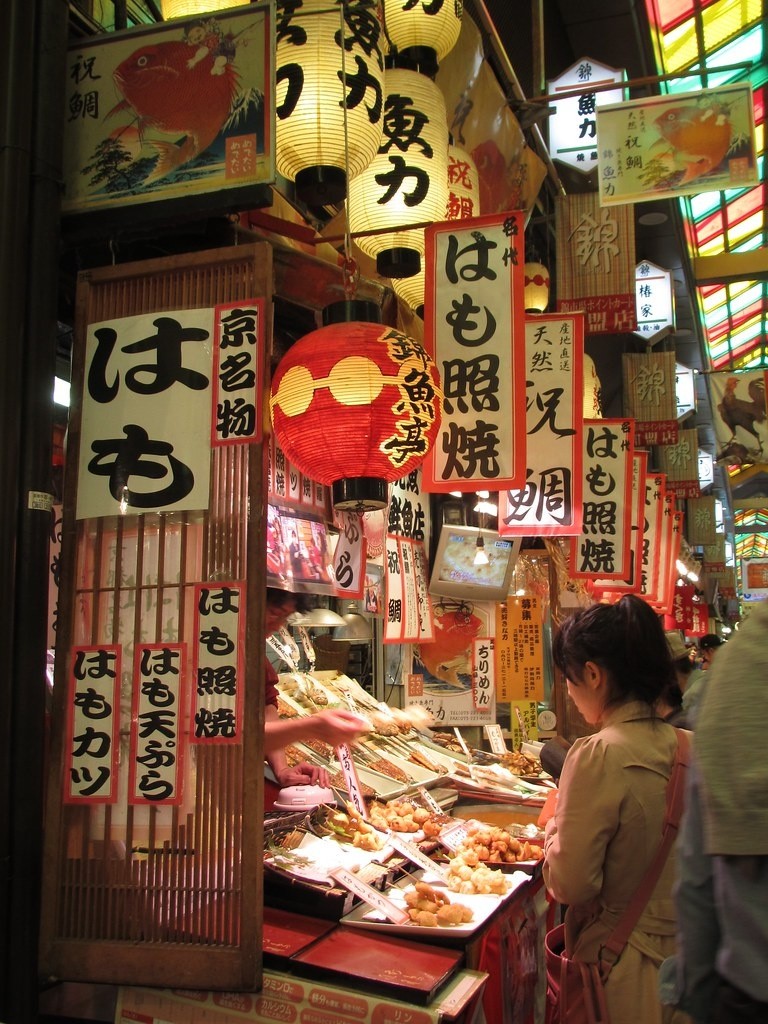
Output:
xmin=538 ymin=595 xmax=698 ymax=1024
xmin=193 ymin=585 xmax=375 ymax=788
xmin=659 ymin=605 xmax=768 ymax=1024
xmin=664 ymin=628 xmax=725 ymax=731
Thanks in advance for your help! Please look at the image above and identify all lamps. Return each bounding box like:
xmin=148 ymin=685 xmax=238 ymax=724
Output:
xmin=331 ymin=599 xmax=375 ymax=642
xmin=289 ymin=595 xmax=347 ymax=629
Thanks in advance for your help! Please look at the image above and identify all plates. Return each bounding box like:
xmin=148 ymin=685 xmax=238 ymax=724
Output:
xmin=274 ymin=672 xmax=552 ymax=806
xmin=339 ymin=837 xmax=545 ymax=937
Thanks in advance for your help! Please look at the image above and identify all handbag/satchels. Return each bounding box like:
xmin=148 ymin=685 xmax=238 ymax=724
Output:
xmin=542 ymin=921 xmax=620 ymax=1024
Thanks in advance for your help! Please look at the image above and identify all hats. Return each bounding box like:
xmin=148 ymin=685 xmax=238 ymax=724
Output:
xmin=699 ymin=634 xmax=726 ymax=647
xmin=664 ymin=631 xmax=691 ymax=661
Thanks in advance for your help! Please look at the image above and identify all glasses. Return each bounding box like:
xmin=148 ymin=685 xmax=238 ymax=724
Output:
xmin=265 ymin=613 xmax=289 ymax=627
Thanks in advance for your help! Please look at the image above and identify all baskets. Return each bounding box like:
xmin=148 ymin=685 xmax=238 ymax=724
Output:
xmin=313 ymin=634 xmax=351 ymax=675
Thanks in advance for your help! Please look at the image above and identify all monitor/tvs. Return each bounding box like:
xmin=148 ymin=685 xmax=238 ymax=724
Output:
xmin=427 ymin=524 xmax=522 ymax=603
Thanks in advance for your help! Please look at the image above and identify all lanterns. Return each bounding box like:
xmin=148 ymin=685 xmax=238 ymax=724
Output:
xmin=390 ymin=132 xmax=481 ymax=320
xmin=267 ymin=320 xmax=444 ymax=518
xmin=275 ymin=0 xmax=384 ymax=206
xmin=583 ymin=353 xmax=603 ymax=418
xmin=162 ymin=0 xmax=251 ymax=22
xmin=679 ymin=538 xmax=702 ymax=580
xmin=384 ymin=0 xmax=463 ymax=72
xmin=524 ymin=253 xmax=550 ymax=313
xmin=343 ymin=67 xmax=449 ymax=278
